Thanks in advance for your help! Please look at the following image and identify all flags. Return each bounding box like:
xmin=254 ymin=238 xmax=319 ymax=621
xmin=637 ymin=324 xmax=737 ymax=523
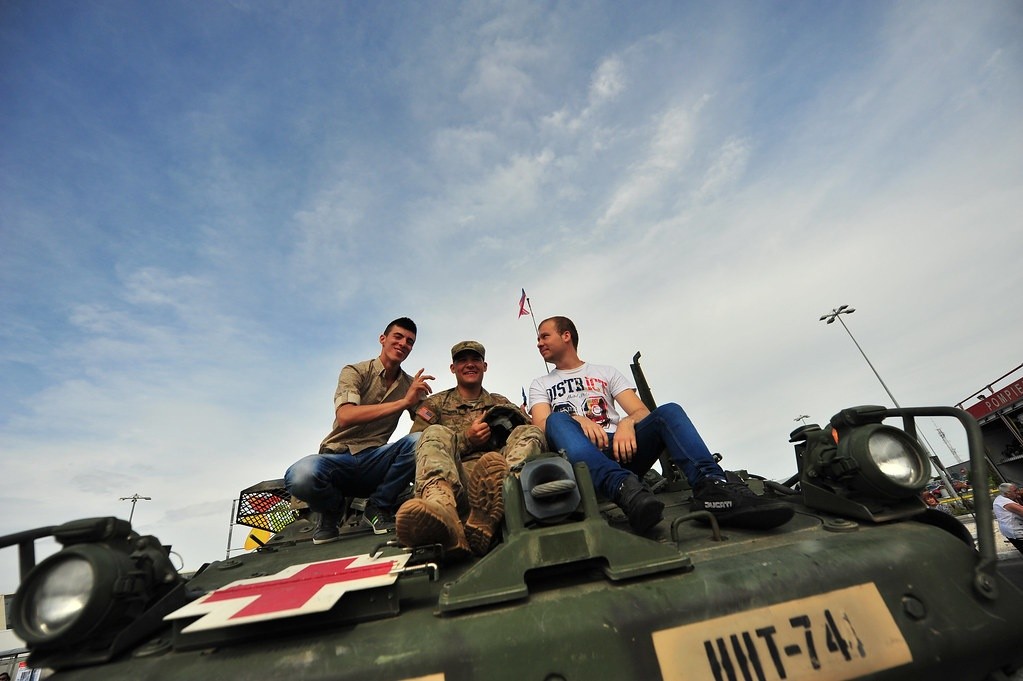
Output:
xmin=518 ymin=289 xmax=529 ymax=319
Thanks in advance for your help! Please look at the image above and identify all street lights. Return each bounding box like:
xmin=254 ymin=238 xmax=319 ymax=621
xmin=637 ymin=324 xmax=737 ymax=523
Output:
xmin=817 ymin=303 xmax=961 ymax=499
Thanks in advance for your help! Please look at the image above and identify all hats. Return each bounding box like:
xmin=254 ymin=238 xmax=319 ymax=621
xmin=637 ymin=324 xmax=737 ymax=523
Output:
xmin=451 ymin=340 xmax=485 ymax=361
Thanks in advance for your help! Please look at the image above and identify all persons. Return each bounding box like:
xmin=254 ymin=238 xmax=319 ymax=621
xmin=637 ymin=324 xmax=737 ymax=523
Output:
xmin=992 ymin=482 xmax=1023 ymax=554
xmin=395 ymin=340 xmax=547 ymax=558
xmin=284 ymin=317 xmax=436 ymax=545
xmin=922 ymin=491 xmax=940 ymax=509
xmin=527 ymin=315 xmax=795 ymax=530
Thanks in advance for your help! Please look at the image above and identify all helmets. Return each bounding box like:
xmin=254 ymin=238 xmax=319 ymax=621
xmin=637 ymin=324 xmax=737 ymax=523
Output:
xmin=478 ymin=404 xmax=533 ymax=452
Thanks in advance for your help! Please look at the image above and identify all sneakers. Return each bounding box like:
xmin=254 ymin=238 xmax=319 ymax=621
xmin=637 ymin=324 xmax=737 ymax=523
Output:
xmin=615 ymin=476 xmax=664 ymax=534
xmin=312 ymin=511 xmax=341 ymax=545
xmin=362 ymin=500 xmax=397 ymax=535
xmin=690 ymin=470 xmax=796 ymax=531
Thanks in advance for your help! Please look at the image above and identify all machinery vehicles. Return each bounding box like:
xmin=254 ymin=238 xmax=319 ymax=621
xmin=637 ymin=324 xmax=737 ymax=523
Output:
xmin=8 ymin=347 xmax=1023 ymax=681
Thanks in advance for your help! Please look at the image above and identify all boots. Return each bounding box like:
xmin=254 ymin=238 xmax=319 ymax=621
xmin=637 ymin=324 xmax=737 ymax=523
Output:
xmin=395 ymin=479 xmax=474 ymax=565
xmin=462 ymin=452 xmax=510 ymax=556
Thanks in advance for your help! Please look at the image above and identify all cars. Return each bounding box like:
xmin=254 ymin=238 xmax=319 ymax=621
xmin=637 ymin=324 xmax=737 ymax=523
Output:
xmin=931 ymin=479 xmax=969 ymax=498
